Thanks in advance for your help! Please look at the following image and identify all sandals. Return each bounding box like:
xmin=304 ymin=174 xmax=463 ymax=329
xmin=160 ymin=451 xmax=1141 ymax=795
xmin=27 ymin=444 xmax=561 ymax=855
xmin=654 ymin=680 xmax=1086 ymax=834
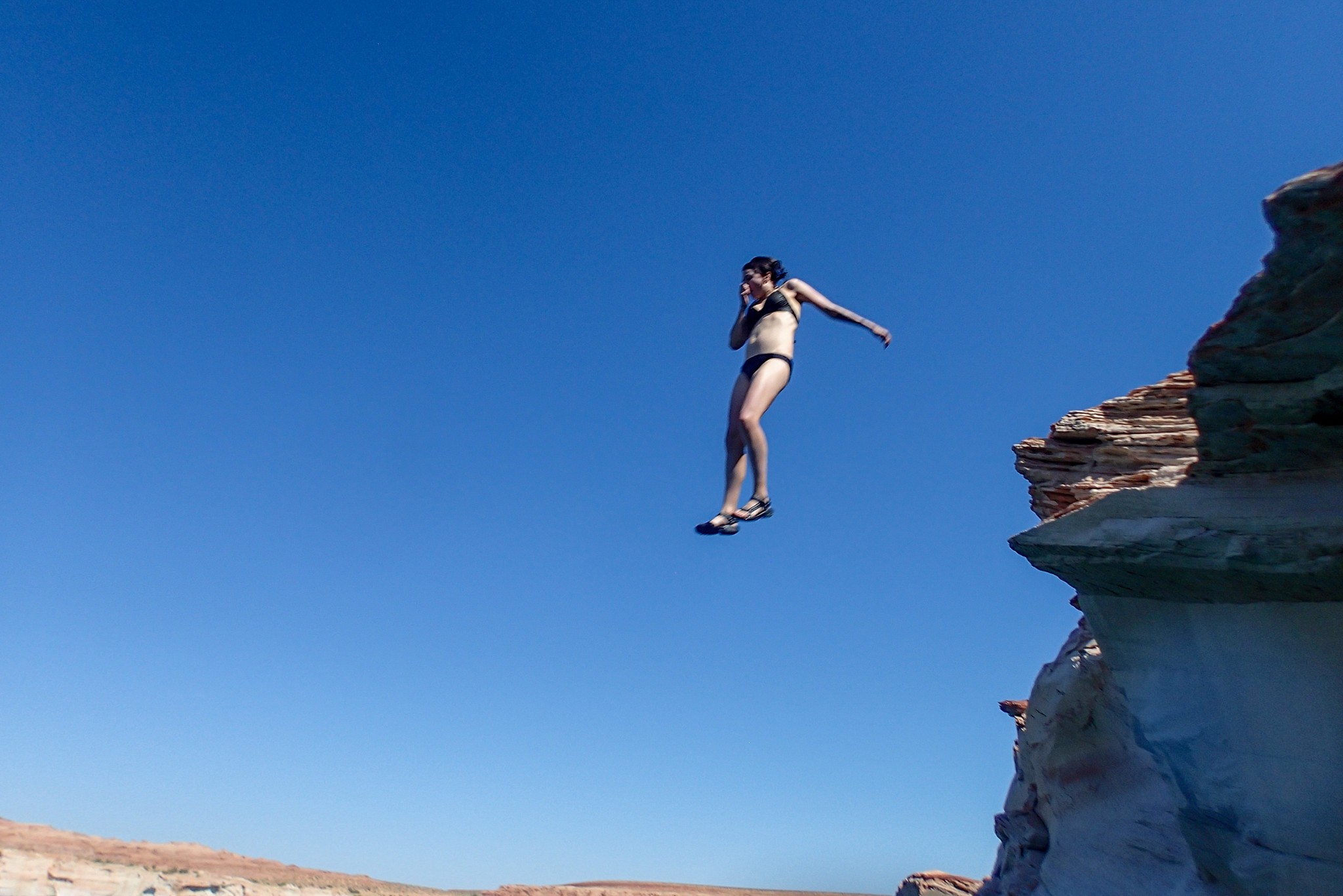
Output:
xmin=731 ymin=496 xmax=775 ymax=522
xmin=695 ymin=512 xmax=740 ymax=535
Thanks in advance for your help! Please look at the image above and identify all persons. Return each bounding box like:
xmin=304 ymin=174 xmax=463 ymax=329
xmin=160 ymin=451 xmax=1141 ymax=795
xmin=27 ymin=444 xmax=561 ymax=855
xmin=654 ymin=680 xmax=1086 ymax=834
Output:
xmin=694 ymin=256 xmax=890 ymax=535
